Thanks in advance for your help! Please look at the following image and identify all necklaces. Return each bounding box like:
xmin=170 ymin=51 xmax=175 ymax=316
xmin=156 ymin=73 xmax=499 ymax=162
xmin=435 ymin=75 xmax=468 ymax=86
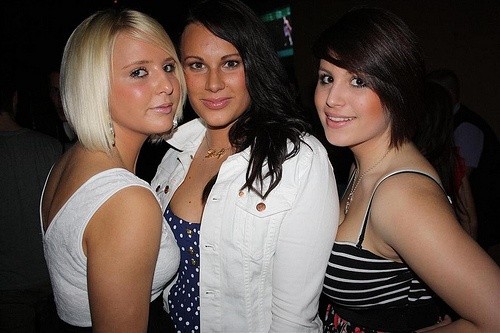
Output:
xmin=344 ymin=148 xmax=390 ymax=214
xmin=205 ymin=129 xmax=235 ymax=160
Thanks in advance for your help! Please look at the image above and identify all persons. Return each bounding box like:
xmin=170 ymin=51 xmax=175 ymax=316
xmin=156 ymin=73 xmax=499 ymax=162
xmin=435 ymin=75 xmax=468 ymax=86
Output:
xmin=419 ymin=68 xmax=500 ymax=266
xmin=0 ymin=62 xmax=78 ymax=333
xmin=301 ymin=8 xmax=500 ymax=333
xmin=39 ymin=9 xmax=188 ymax=333
xmin=150 ymin=0 xmax=340 ymax=333
